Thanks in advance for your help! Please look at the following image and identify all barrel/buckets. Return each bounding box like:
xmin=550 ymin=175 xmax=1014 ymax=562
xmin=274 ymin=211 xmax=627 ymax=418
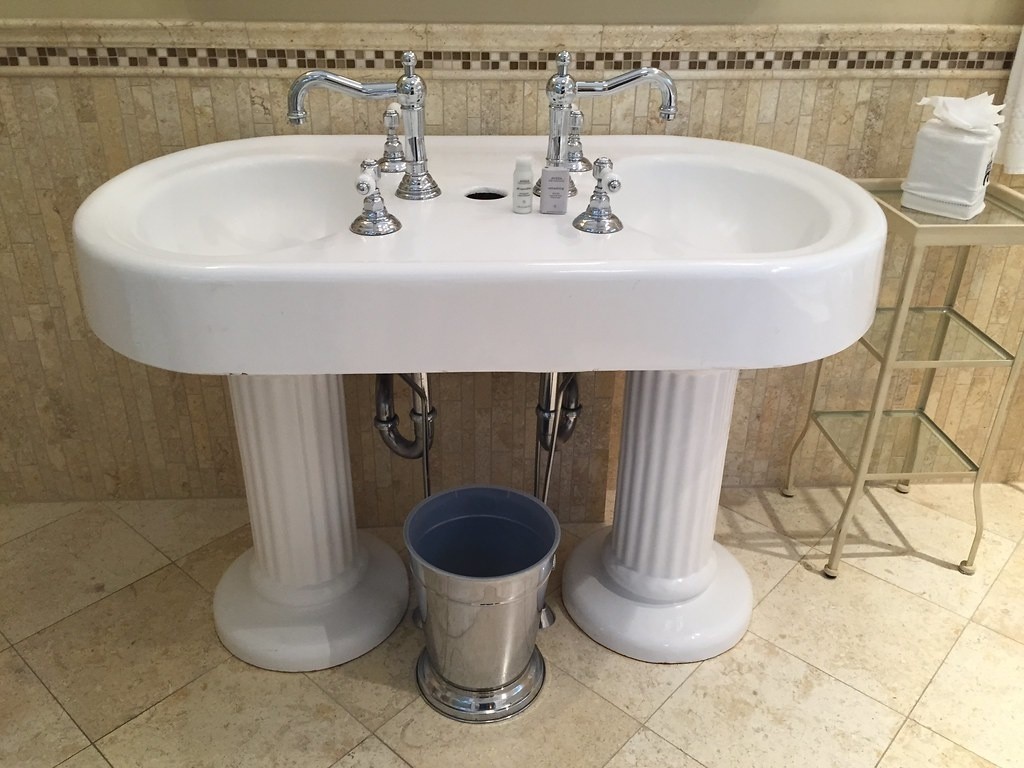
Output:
xmin=402 ymin=485 xmax=560 ymax=724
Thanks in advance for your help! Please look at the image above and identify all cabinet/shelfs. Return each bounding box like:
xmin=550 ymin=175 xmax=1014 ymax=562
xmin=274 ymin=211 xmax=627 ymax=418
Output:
xmin=780 ymin=176 xmax=1024 ymax=579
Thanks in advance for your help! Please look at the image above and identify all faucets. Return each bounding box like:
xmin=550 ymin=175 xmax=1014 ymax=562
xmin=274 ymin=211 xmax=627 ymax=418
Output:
xmin=282 ymin=52 xmax=444 ymax=202
xmin=546 ymin=51 xmax=679 ymax=172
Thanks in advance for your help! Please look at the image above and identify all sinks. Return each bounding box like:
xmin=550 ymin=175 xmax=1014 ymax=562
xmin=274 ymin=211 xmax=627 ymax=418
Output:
xmin=599 ymin=127 xmax=888 ymax=372
xmin=71 ymin=135 xmax=379 ymax=377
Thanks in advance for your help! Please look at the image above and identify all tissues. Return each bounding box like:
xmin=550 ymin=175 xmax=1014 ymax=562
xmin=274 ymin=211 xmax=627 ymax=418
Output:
xmin=898 ymin=90 xmax=1008 ymax=223
